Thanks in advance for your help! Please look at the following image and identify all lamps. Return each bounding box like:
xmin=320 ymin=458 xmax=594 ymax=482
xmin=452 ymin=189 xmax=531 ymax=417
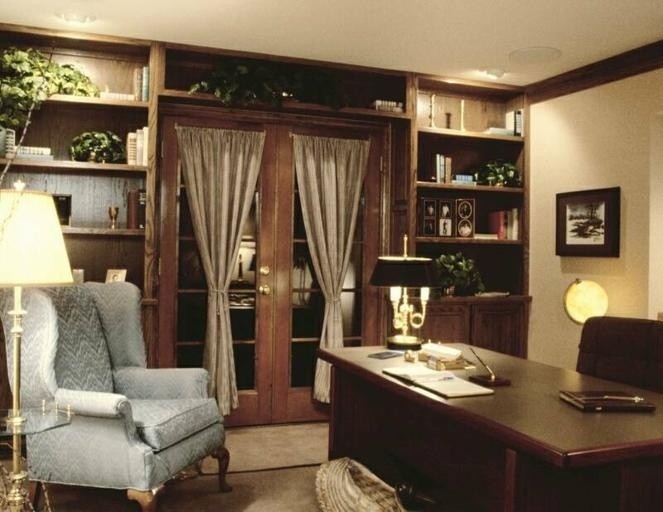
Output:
xmin=563 ymin=277 xmax=609 ymax=323
xmin=369 ymin=235 xmax=429 ymax=350
xmin=1 ymin=189 xmax=72 ymax=512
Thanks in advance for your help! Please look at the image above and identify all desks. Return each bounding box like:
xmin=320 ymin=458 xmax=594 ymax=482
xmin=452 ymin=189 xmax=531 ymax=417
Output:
xmin=317 ymin=343 xmax=663 ymax=512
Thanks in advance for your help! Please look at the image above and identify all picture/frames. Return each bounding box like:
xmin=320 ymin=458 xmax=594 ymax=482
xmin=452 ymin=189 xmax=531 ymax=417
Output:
xmin=555 ymin=185 xmax=620 ymax=260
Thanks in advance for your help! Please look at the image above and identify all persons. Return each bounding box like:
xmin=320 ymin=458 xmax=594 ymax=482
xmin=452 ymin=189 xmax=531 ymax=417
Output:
xmin=442 ymin=220 xmax=448 ymax=235
xmin=427 ymin=204 xmax=434 ymax=217
xmin=427 ymin=221 xmax=434 ymax=233
xmin=443 ymin=202 xmax=448 ymax=217
xmin=459 ymin=222 xmax=471 ymax=235
xmin=463 ymin=204 xmax=468 ymax=216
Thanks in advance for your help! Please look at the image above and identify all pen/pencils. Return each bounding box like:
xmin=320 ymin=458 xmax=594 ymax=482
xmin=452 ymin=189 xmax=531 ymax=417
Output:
xmin=469 ymin=346 xmax=494 ymax=375
xmin=603 ymin=395 xmax=634 ymax=399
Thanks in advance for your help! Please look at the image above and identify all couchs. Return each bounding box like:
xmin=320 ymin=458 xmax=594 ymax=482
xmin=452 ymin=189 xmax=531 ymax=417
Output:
xmin=0 ymin=279 xmax=231 ymax=512
xmin=576 ymin=317 xmax=663 ymax=392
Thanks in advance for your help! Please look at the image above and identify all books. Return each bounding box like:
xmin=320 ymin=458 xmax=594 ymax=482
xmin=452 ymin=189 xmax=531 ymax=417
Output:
xmin=483 ymin=110 xmax=522 ymax=136
xmin=15 ymin=142 xmax=54 ymax=160
xmin=475 ymin=207 xmax=519 ymax=238
xmin=127 ymin=125 xmax=147 ymax=165
xmin=127 ymin=189 xmax=146 ymax=228
xmin=370 ymin=99 xmax=403 ymax=112
xmin=382 ymin=363 xmax=496 ymax=399
xmin=100 ymin=66 xmax=150 ymax=101
xmin=429 ymin=153 xmax=452 ymax=184
xmin=557 ymin=388 xmax=657 ymax=414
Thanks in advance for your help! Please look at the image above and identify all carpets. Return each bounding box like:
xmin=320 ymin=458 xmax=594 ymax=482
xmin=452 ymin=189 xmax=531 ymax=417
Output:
xmin=4 ymin=421 xmax=330 ymax=512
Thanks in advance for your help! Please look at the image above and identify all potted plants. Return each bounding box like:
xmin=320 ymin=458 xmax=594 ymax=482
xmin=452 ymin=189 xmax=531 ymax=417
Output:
xmin=481 ymin=159 xmax=520 ymax=187
xmin=430 ymin=251 xmax=486 ymax=297
xmin=71 ymin=132 xmax=123 ymax=165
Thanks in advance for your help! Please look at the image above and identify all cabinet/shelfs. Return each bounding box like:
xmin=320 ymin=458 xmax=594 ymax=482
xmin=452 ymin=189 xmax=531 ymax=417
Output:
xmin=411 ymin=73 xmax=529 ymax=294
xmin=0 ymin=23 xmax=159 ymax=290
xmin=388 ymin=298 xmax=532 ymax=358
